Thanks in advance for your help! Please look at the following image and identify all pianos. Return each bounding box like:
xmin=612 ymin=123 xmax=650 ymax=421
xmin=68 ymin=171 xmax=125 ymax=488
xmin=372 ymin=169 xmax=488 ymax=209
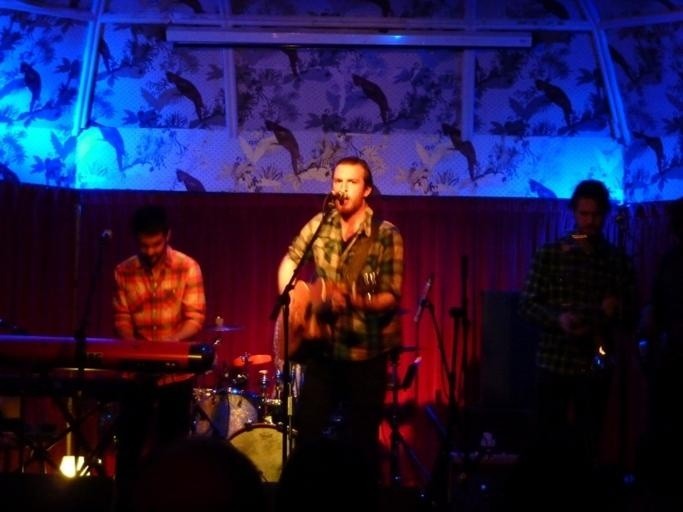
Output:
xmin=1 ymin=335 xmax=214 ymax=374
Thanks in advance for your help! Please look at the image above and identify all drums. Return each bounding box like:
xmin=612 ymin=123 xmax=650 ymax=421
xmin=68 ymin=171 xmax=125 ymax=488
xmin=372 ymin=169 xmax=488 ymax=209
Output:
xmin=261 ymin=399 xmax=295 ymax=429
xmin=226 ymin=421 xmax=298 ymax=483
xmin=193 ymin=389 xmax=265 ymax=441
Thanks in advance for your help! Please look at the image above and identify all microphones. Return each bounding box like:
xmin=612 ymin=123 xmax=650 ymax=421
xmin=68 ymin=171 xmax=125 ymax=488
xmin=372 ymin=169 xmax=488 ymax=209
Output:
xmin=414 ymin=276 xmax=432 ymax=323
xmin=616 ymin=215 xmax=625 ymax=231
xmin=100 ymin=229 xmax=112 ymax=253
xmin=330 ymin=191 xmax=345 ymax=202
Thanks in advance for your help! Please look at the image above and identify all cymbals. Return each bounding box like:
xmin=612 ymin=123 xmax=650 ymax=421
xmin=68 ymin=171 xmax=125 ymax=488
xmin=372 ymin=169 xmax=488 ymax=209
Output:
xmin=208 ymin=322 xmax=247 ymax=332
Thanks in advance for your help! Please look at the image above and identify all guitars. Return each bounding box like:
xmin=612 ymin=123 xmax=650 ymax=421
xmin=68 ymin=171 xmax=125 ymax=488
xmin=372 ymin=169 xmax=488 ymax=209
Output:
xmin=272 ymin=270 xmax=382 ymax=364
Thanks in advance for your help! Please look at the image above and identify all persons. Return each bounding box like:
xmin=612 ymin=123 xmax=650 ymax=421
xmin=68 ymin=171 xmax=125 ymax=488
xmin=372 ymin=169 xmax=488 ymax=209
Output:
xmin=109 ymin=207 xmax=208 ymax=489
xmin=518 ymin=178 xmax=682 ymax=470
xmin=277 ymin=158 xmax=404 ymax=500
